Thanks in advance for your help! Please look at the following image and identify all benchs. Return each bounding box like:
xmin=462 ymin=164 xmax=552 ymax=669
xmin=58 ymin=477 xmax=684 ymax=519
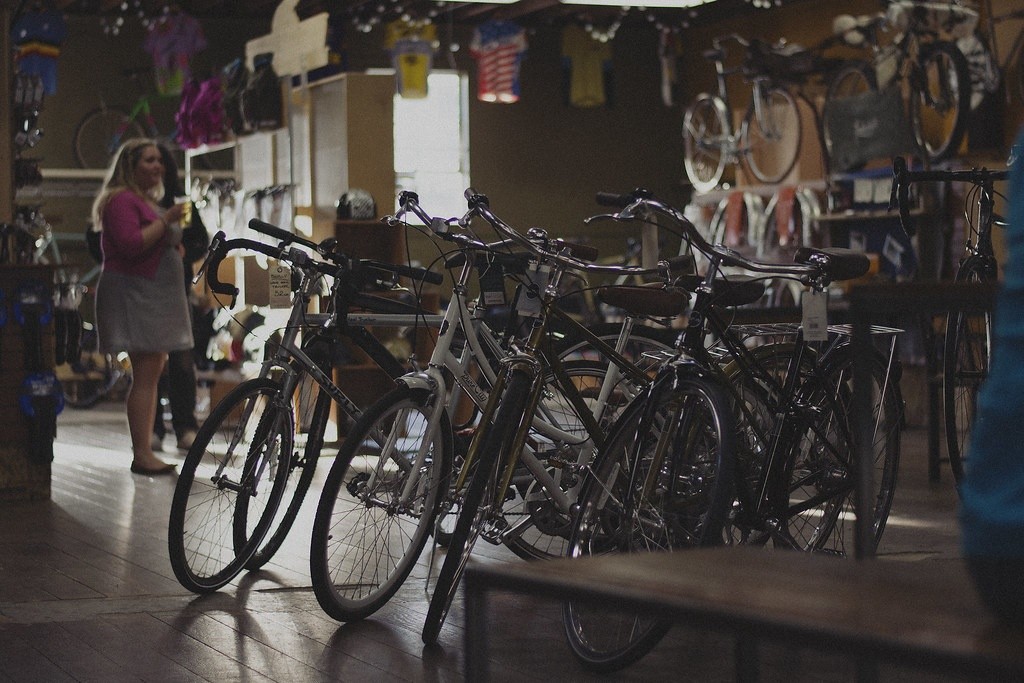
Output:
xmin=459 ymin=542 xmax=1024 ymax=683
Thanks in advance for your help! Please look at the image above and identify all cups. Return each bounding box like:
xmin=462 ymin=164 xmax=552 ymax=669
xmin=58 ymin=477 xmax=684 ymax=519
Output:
xmin=175 ymin=196 xmax=193 ymax=228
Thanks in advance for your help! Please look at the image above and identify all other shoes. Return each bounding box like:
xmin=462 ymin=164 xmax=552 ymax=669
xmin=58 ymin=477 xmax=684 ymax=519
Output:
xmin=150 ymin=432 xmax=161 ymax=450
xmin=178 ymin=430 xmax=197 ymax=448
xmin=130 ymin=459 xmax=175 ymax=474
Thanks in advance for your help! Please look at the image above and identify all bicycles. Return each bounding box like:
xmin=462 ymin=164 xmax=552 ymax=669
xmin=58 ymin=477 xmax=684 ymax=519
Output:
xmin=886 ymin=155 xmax=1009 ymax=498
xmin=166 ymin=188 xmax=909 ymax=675
xmin=820 ymin=0 xmax=972 ymax=172
xmin=681 ymin=34 xmax=803 ymax=194
xmin=73 ymin=65 xmax=184 ymax=169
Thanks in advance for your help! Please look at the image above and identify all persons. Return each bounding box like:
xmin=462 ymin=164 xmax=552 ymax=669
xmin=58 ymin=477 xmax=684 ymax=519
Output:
xmin=87 ymin=139 xmax=209 ymax=474
xmin=959 ymin=130 xmax=1024 ymax=630
